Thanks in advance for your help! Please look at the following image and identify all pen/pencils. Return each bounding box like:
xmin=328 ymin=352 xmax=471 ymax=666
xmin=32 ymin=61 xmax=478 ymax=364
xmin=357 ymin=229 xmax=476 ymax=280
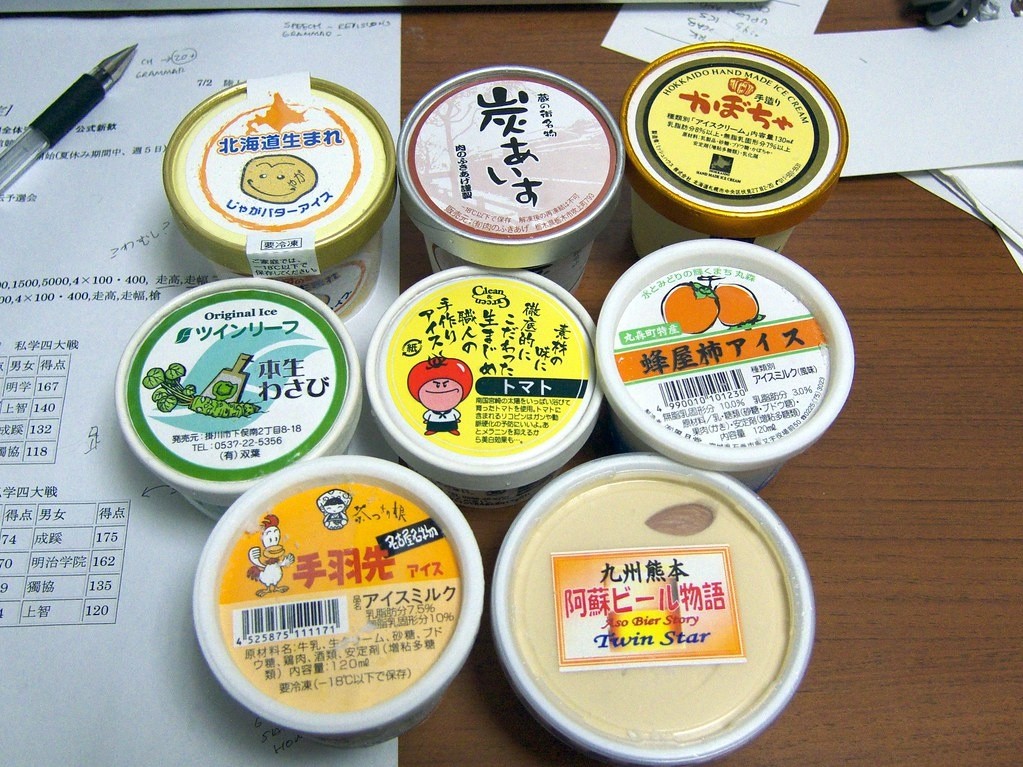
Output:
xmin=1 ymin=42 xmax=135 ymax=197
